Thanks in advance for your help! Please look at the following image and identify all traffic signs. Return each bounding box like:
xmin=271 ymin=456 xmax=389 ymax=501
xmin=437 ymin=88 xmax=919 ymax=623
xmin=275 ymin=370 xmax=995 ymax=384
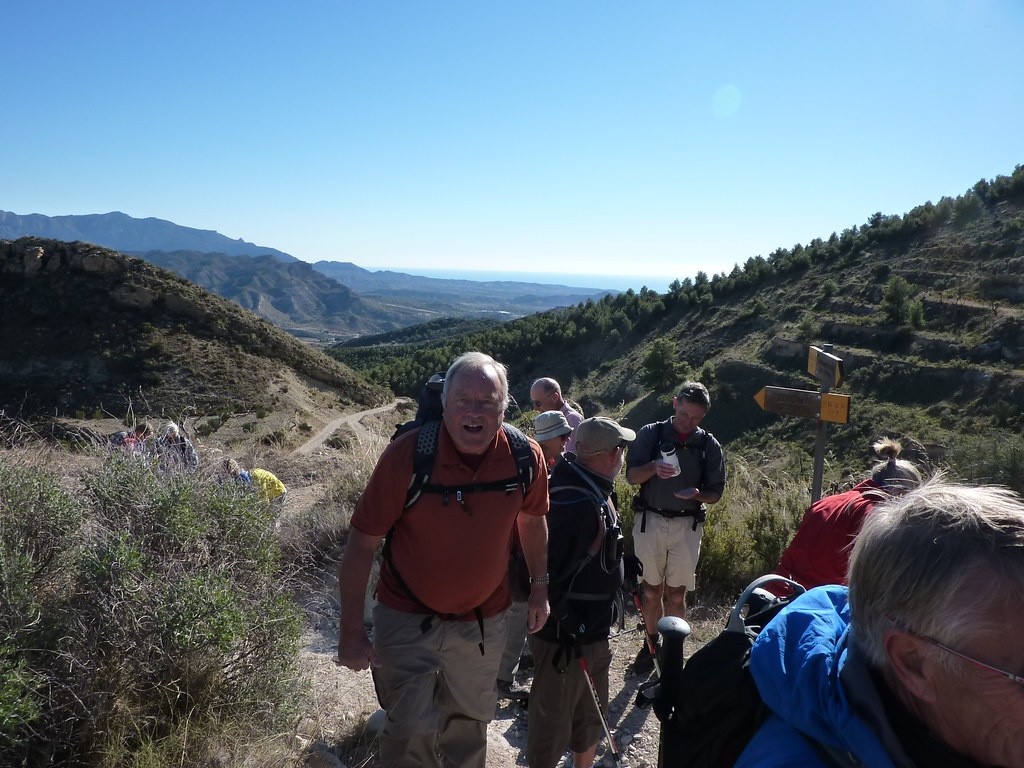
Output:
xmin=754 ymin=386 xmax=851 ymax=425
xmin=807 ymin=345 xmax=845 ymax=388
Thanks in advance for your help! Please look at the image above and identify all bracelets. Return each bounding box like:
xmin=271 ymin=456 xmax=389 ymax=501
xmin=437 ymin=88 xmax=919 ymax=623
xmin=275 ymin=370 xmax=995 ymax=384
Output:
xmin=529 ymin=574 xmax=550 ymax=584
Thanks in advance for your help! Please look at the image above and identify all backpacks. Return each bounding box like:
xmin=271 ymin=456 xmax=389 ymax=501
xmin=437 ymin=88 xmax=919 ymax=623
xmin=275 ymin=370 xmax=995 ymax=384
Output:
xmin=656 ymin=572 xmax=857 ymax=768
xmin=390 ymin=371 xmax=536 ymax=512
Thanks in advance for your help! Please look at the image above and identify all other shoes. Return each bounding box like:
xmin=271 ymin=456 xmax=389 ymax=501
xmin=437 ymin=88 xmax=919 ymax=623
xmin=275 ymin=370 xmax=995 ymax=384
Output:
xmin=634 ymin=635 xmax=660 ymax=673
xmin=497 ymin=679 xmax=529 ymax=699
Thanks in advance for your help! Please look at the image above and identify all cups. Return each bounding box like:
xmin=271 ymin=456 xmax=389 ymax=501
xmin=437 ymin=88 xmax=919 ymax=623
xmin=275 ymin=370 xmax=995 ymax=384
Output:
xmin=660 ymin=443 xmax=681 ymax=477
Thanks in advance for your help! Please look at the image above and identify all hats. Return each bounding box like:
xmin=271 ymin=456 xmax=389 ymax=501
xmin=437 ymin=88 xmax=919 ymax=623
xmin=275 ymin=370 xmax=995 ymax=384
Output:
xmin=574 ymin=416 xmax=637 ymax=456
xmin=534 ymin=410 xmax=574 ymax=441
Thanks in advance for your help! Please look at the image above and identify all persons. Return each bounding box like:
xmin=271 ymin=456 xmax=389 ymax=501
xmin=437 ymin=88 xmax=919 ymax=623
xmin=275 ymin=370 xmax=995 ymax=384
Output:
xmin=337 ymin=351 xmax=726 ymax=768
xmin=108 ymin=421 xmax=287 ymax=527
xmin=736 ymin=436 xmax=1024 ymax=768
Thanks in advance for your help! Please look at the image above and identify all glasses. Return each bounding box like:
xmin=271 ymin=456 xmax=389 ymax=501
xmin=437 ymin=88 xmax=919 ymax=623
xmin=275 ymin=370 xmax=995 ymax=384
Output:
xmin=530 ymin=391 xmax=559 ymax=406
xmin=673 ymin=388 xmax=709 ymax=403
